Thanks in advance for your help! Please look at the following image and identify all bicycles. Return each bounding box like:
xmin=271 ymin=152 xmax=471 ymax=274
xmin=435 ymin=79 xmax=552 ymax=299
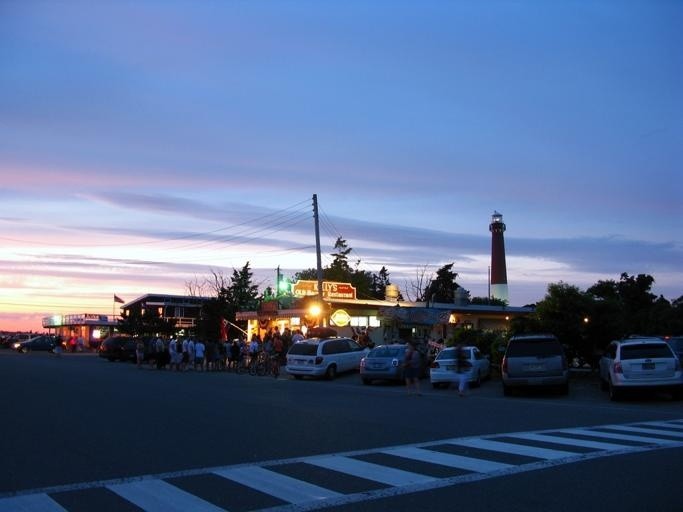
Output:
xmin=231 ymin=349 xmax=286 ymax=381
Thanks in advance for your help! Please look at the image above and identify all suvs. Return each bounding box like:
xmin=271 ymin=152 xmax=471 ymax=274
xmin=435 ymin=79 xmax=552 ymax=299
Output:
xmin=498 ymin=333 xmax=570 ymax=397
xmin=594 ymin=331 xmax=682 ymax=401
xmin=658 ymin=335 xmax=682 ymax=361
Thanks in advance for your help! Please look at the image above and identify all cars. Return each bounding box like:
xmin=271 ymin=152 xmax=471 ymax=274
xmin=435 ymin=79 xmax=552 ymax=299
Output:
xmin=356 ymin=342 xmax=411 ymax=386
xmin=11 ymin=336 xmax=55 ymax=354
xmin=427 ymin=345 xmax=491 ymax=389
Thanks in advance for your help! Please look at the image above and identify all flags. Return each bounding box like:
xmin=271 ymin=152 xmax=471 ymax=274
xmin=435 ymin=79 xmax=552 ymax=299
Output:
xmin=113 ymin=294 xmax=125 ymax=304
xmin=218 ymin=314 xmax=228 ymax=342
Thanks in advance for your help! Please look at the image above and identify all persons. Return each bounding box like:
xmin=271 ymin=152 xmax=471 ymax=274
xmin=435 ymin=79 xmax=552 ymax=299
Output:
xmin=52 ymin=333 xmax=84 ymax=358
xmin=132 ymin=325 xmax=376 ymax=380
xmin=397 ymin=342 xmax=423 ymax=398
xmin=452 ymin=343 xmax=473 ymax=397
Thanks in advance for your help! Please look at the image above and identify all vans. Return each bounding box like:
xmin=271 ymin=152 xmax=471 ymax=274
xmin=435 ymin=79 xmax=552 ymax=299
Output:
xmin=282 ymin=326 xmax=370 ymax=383
xmin=11 ymin=333 xmax=37 ymax=342
xmin=96 ymin=334 xmax=156 ymax=363
